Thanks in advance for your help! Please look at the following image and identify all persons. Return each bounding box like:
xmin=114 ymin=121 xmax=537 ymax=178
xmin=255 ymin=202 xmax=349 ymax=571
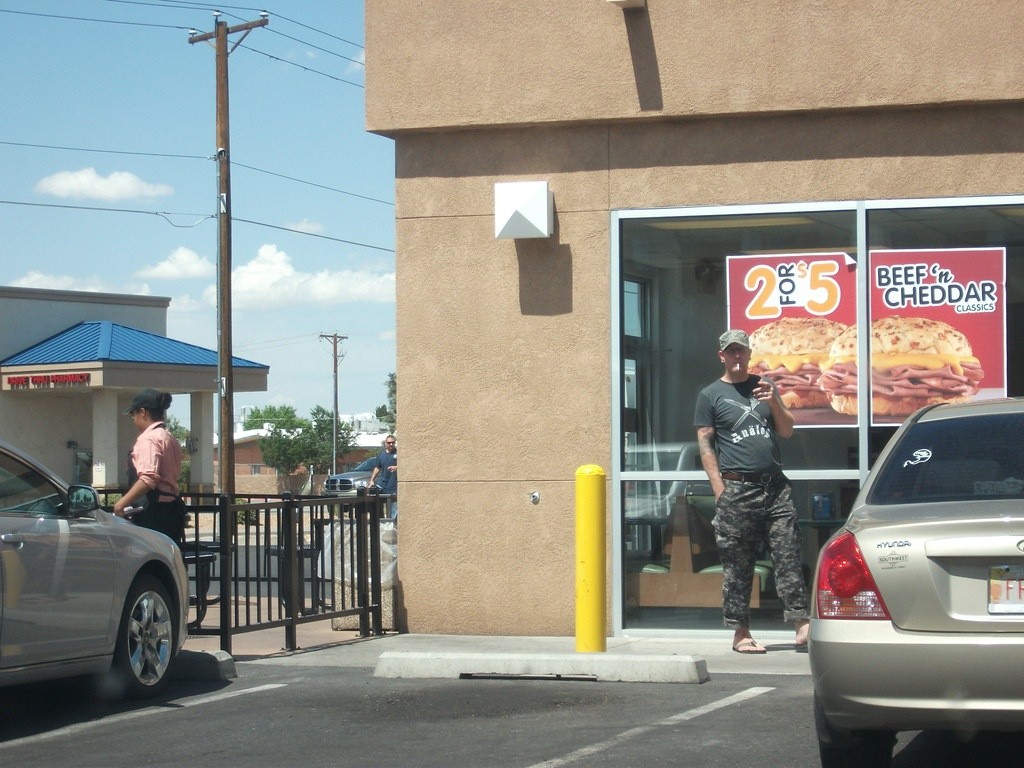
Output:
xmin=113 ymin=388 xmax=186 ymax=549
xmin=369 ymin=434 xmax=397 ymax=525
xmin=693 ymin=329 xmax=812 ymax=655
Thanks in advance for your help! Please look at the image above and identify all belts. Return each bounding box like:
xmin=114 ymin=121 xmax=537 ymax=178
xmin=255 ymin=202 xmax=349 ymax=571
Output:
xmin=722 ymin=467 xmax=782 ymax=482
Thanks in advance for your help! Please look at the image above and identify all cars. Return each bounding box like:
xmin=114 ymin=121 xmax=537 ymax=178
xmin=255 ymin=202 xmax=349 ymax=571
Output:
xmin=323 ymin=456 xmax=381 ymax=514
xmin=622 ymin=440 xmax=710 ymax=555
xmin=0 ymin=436 xmax=191 ymax=700
xmin=804 ymin=397 xmax=1024 ymax=768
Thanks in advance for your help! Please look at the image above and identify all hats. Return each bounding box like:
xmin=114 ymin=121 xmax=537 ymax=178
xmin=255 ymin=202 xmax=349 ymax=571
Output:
xmin=122 ymin=389 xmax=161 ymax=416
xmin=719 ymin=329 xmax=750 ymax=352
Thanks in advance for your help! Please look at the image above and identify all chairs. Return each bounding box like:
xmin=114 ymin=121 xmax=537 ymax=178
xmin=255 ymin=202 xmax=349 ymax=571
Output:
xmin=909 ymin=457 xmax=1007 ymax=498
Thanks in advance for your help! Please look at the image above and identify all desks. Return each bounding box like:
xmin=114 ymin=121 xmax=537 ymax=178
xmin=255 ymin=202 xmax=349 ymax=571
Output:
xmin=796 ymin=517 xmax=849 ymax=555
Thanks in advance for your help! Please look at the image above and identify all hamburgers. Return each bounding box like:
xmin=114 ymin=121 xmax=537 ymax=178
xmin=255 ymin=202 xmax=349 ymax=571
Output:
xmin=744 ymin=313 xmax=986 ymax=419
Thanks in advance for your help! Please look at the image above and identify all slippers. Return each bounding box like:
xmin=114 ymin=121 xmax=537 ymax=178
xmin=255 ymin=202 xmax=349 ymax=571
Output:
xmin=733 ymin=640 xmax=808 ymax=654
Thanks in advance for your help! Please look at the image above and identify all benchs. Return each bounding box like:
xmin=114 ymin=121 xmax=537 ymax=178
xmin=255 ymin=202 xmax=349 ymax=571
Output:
xmin=622 ymin=495 xmax=774 ymax=611
